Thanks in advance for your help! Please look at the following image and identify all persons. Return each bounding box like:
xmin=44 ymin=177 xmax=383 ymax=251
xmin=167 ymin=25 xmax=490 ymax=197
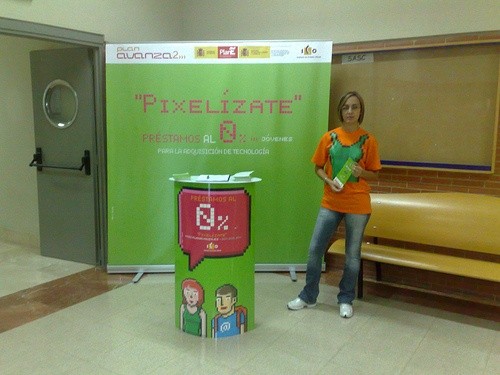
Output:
xmin=286 ymin=91 xmax=383 ymax=320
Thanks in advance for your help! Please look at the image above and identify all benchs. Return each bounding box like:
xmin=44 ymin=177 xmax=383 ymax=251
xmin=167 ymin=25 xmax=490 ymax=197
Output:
xmin=324 ymin=191 xmax=500 ymax=309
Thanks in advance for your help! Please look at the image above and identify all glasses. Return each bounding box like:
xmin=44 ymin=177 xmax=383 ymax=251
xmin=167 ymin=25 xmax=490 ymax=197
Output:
xmin=340 ymin=104 xmax=360 ymax=111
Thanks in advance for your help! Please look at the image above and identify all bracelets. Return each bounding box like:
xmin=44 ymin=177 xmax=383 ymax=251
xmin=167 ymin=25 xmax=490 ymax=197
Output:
xmin=323 ymin=177 xmax=328 ymax=181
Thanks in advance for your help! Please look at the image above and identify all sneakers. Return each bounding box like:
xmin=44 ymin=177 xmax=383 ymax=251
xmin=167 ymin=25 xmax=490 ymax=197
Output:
xmin=288 ymin=298 xmax=317 ymax=309
xmin=340 ymin=303 xmax=352 ymax=318
